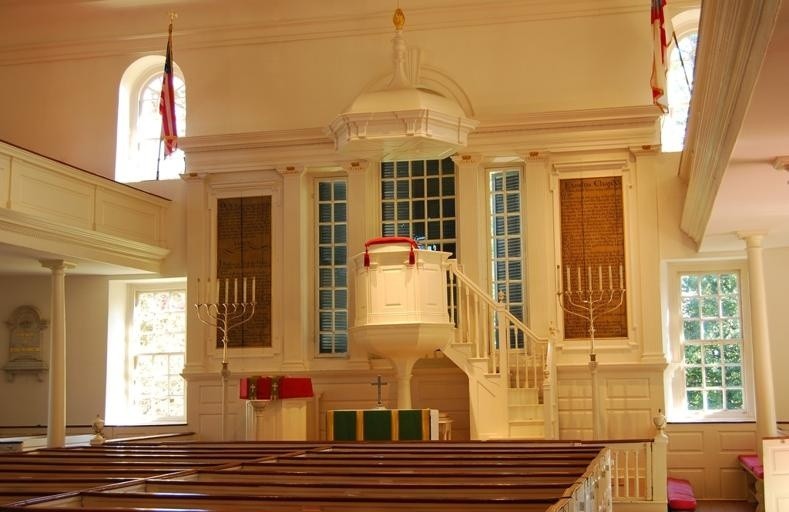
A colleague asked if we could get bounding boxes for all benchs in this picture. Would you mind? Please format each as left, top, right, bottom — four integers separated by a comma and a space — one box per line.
667, 477, 697, 512
0, 438, 614, 512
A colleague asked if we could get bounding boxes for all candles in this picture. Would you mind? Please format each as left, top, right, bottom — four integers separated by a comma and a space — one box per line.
552, 263, 626, 291
195, 277, 256, 303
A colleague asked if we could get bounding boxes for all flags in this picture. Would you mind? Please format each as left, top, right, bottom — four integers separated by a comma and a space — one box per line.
646, 1, 674, 119
157, 20, 179, 161
